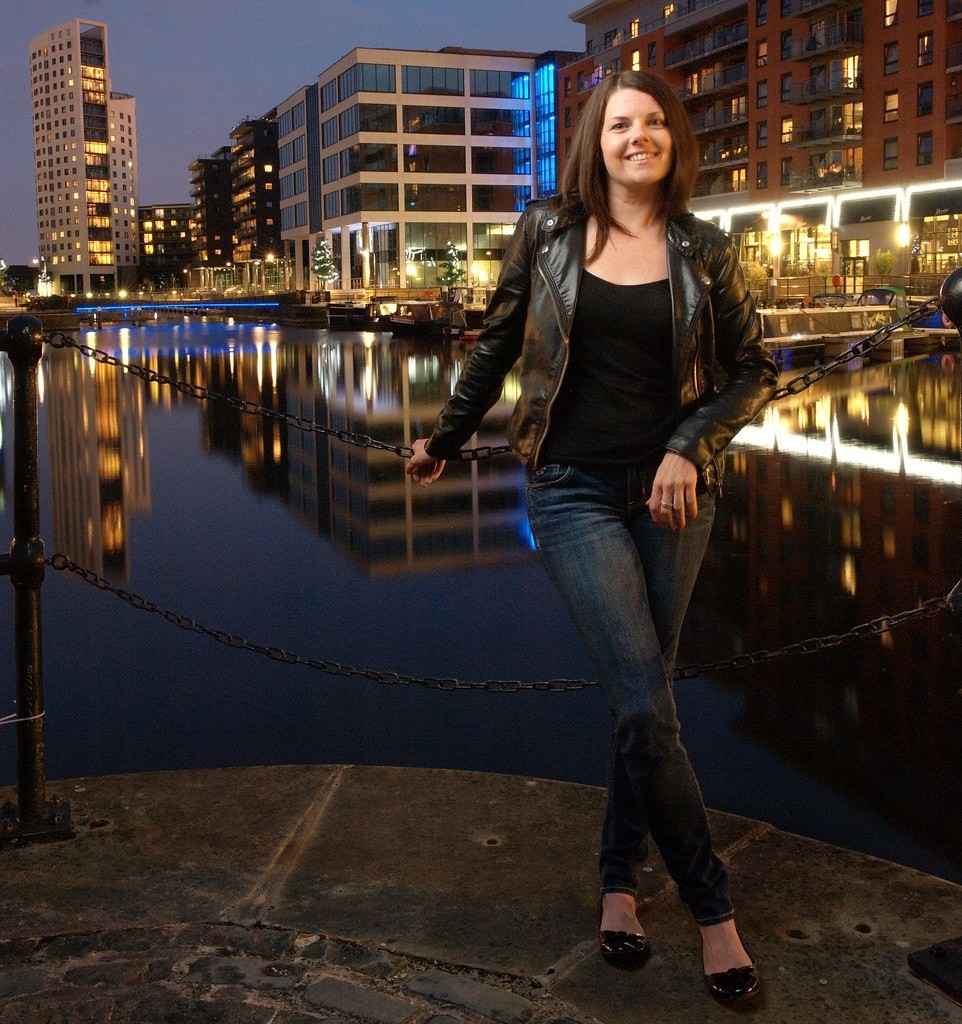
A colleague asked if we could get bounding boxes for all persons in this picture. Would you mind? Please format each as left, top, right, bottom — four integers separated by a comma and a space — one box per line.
406, 70, 779, 999
291, 290, 326, 304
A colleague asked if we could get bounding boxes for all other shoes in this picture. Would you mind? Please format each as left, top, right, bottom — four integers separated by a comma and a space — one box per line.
596, 894, 645, 959
701, 926, 761, 1002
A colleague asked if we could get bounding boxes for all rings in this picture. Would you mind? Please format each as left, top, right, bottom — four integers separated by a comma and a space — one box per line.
661, 501, 673, 509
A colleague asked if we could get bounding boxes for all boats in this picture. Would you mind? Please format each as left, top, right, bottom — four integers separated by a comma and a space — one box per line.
324, 300, 368, 331
388, 300, 466, 340
803, 287, 914, 331
